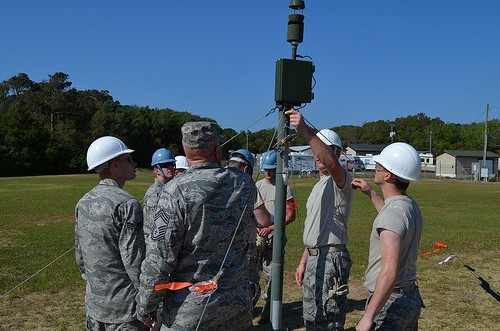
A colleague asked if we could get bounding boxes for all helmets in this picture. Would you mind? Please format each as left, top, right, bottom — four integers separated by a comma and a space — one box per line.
230, 149, 254, 176
316, 129, 343, 150
373, 142, 421, 182
86, 136, 135, 171
261, 150, 277, 169
174, 156, 189, 170
151, 148, 177, 166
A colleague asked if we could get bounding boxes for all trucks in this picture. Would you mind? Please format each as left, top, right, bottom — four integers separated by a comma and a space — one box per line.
338, 155, 365, 171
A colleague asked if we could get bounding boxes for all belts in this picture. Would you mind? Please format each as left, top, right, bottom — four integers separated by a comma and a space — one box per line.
368, 281, 415, 293
307, 245, 345, 255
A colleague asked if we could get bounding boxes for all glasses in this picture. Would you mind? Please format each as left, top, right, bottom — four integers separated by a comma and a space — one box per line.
376, 163, 386, 172
160, 163, 173, 168
125, 156, 132, 162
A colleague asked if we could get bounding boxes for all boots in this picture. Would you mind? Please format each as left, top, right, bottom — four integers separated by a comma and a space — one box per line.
256, 302, 270, 324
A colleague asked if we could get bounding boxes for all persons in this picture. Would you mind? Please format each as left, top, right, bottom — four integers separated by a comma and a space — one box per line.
135, 122, 259, 331
285, 110, 354, 331
174, 156, 189, 177
142, 148, 177, 242
351, 143, 426, 331
253, 150, 296, 322
227, 148, 272, 228
74, 136, 149, 331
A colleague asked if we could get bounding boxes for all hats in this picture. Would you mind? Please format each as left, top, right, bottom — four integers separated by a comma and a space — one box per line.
181, 122, 225, 149
